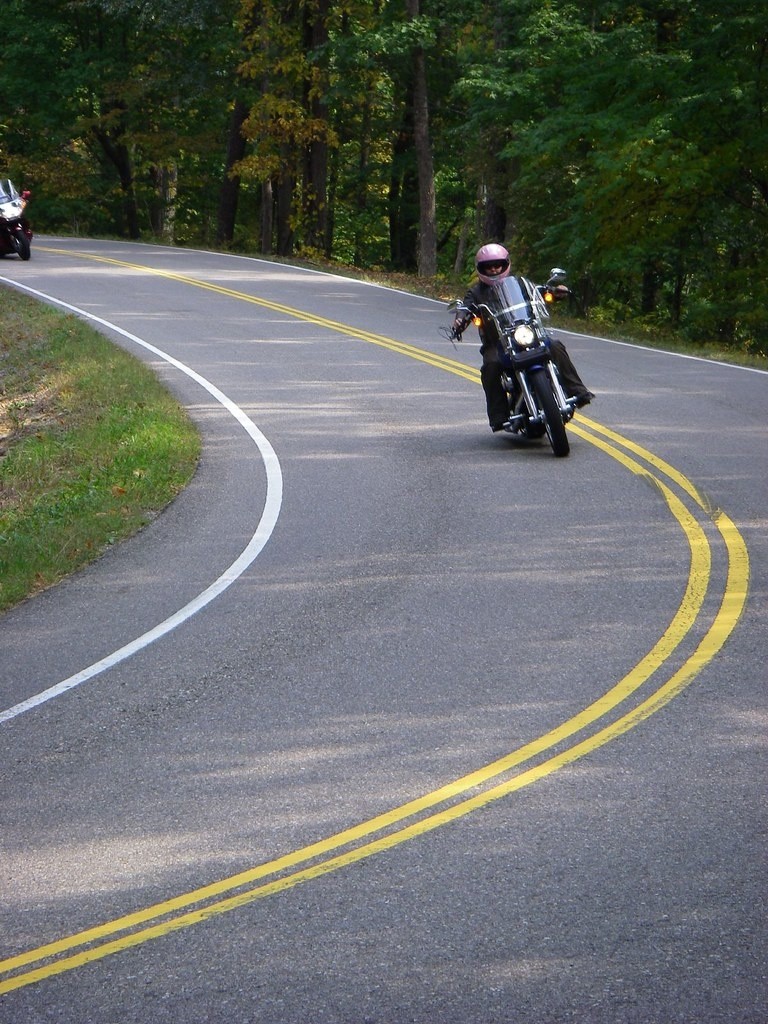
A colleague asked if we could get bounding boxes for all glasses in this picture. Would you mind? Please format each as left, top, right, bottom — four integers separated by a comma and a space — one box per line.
483, 262, 502, 270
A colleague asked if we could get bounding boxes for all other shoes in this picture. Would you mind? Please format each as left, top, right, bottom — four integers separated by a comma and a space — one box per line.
489, 417, 508, 432
575, 391, 592, 409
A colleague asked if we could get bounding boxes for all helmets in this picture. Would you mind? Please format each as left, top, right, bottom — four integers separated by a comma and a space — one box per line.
475, 244, 511, 285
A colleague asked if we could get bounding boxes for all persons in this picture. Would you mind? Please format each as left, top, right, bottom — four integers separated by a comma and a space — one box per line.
453, 244, 590, 429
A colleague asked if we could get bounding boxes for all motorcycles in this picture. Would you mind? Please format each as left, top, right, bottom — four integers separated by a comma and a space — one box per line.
0, 177, 33, 262
447, 268, 591, 458
